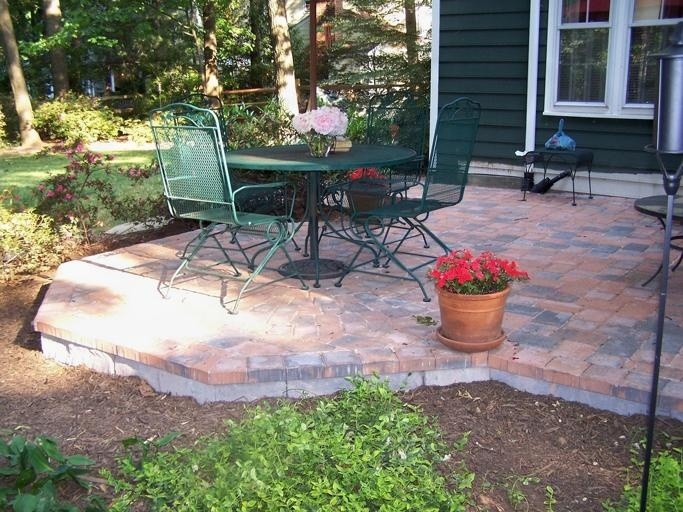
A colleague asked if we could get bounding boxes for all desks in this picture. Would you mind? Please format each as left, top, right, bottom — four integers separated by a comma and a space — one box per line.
635, 195, 683, 287
229, 145, 417, 278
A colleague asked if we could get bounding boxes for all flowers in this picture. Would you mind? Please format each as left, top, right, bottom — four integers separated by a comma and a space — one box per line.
426, 243, 531, 296
293, 105, 351, 135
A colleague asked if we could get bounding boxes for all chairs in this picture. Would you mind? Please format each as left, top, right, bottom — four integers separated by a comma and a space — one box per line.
150, 104, 309, 313
334, 99, 483, 301
311, 92, 428, 267
169, 94, 303, 269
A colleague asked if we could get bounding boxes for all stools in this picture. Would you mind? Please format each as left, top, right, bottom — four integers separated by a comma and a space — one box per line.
518, 148, 593, 205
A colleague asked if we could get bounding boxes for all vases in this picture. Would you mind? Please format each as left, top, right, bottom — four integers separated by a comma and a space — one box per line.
309, 133, 331, 157
434, 288, 510, 353
